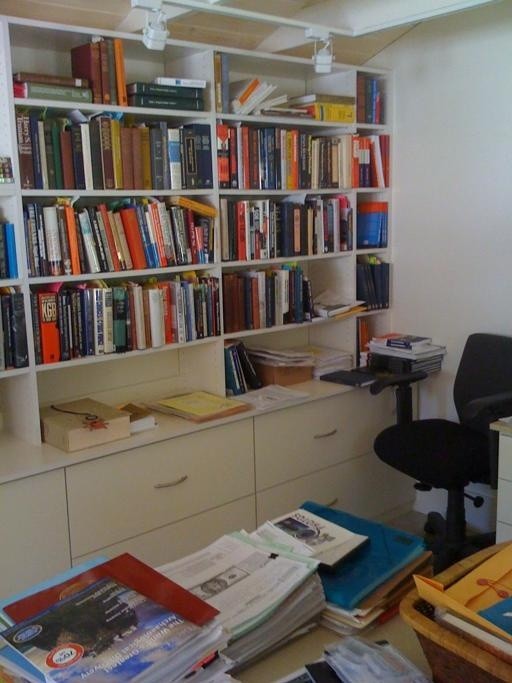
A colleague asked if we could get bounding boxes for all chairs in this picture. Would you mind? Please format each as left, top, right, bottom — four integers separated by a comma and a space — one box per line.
379, 333, 512, 571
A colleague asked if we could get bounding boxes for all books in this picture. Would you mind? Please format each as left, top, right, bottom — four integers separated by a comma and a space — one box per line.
220, 195, 353, 260
1, 293, 29, 369
31, 272, 221, 364
356, 77, 385, 124
0, 500, 434, 683
358, 317, 445, 372
24, 198, 216, 277
216, 124, 390, 189
1, 223, 18, 279
224, 263, 350, 333
357, 202, 388, 249
357, 262, 389, 309
16, 111, 213, 190
214, 53, 354, 122
40, 399, 156, 451
13, 39, 206, 111
222, 338, 353, 395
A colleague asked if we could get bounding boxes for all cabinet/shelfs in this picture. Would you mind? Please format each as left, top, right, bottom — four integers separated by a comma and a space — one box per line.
2, 14, 419, 630
